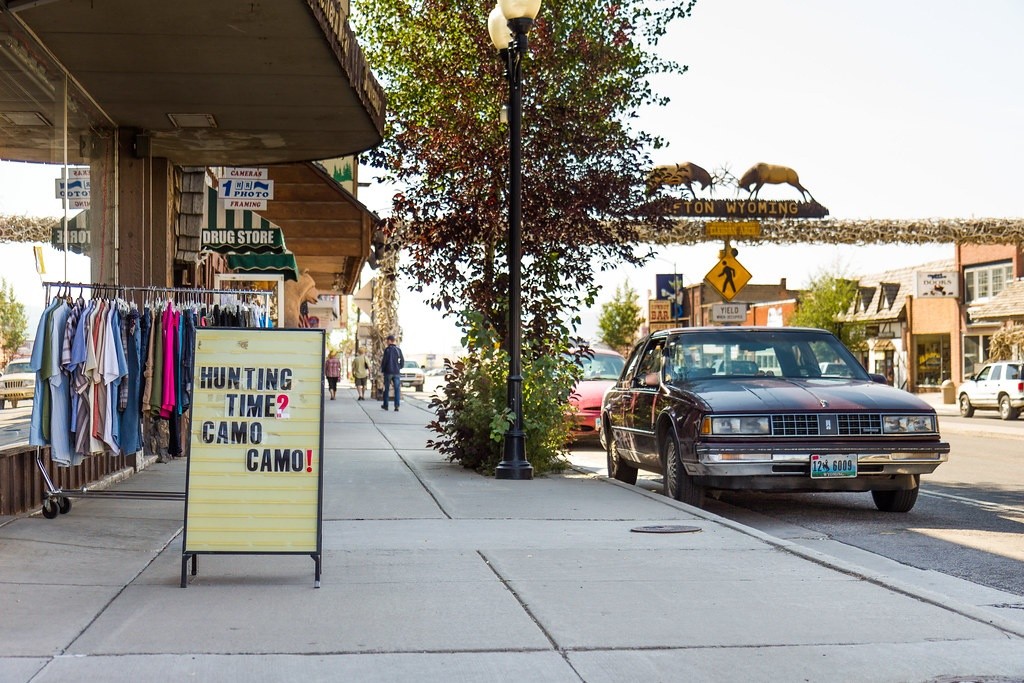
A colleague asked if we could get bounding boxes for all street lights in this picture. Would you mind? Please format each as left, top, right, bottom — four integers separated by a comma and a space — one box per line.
486, 0, 547, 481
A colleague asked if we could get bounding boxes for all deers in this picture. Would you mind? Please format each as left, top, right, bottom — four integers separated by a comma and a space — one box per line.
721, 162, 818, 203
643, 162, 728, 200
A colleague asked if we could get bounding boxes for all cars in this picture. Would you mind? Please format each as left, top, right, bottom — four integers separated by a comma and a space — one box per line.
545, 346, 658, 446
600, 325, 952, 514
396, 360, 426, 392
426, 367, 447, 377
956, 360, 1024, 421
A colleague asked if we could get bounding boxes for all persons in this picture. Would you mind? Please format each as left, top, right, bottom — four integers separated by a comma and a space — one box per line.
325, 350, 341, 399
351, 347, 370, 401
381, 335, 405, 411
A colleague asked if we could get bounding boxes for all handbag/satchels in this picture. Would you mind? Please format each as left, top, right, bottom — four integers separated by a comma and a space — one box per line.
364, 357, 369, 369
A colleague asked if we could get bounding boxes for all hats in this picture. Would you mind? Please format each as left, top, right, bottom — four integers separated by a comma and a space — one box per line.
385, 335, 395, 340
329, 351, 338, 357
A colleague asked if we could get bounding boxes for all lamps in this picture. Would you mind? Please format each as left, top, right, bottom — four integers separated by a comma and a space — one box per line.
333, 274, 341, 281
332, 282, 339, 291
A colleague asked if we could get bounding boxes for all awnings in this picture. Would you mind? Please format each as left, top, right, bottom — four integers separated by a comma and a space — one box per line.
226, 248, 298, 283
203, 183, 286, 255
51, 210, 90, 254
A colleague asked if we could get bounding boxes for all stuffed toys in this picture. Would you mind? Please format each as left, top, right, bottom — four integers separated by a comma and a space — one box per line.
283, 268, 322, 328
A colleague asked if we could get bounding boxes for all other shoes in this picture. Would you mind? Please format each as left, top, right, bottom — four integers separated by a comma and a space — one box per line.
358, 397, 361, 400
381, 405, 388, 410
331, 397, 334, 400
394, 405, 398, 411
362, 397, 365, 400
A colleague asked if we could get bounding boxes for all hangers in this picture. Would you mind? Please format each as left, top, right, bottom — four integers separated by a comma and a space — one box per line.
44, 280, 274, 315
